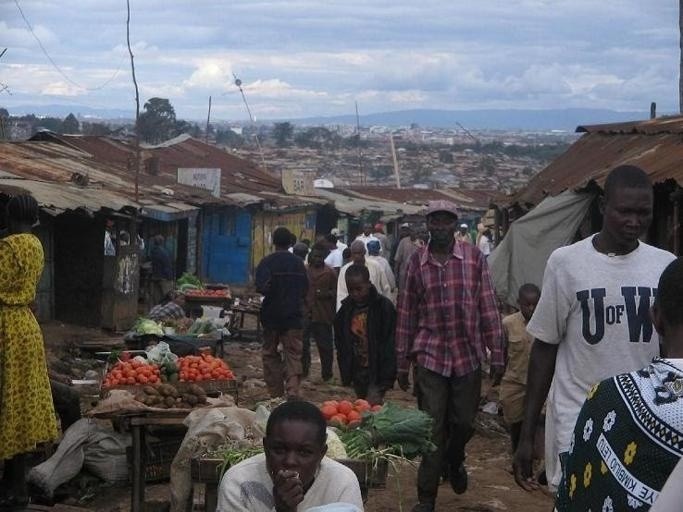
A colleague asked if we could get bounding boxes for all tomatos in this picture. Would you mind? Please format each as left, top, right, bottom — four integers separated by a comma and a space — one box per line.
186, 290, 228, 298
315, 399, 382, 424
102, 352, 162, 386
175, 353, 238, 382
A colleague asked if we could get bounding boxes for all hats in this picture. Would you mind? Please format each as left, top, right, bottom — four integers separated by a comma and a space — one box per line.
424, 199, 460, 220
401, 222, 415, 233
367, 241, 381, 252
374, 223, 384, 232
342, 248, 351, 259
293, 243, 306, 257
330, 227, 344, 238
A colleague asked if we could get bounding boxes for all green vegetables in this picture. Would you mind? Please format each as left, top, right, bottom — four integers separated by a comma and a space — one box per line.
339, 402, 438, 461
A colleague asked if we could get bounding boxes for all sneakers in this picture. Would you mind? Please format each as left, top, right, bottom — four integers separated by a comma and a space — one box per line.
410, 499, 436, 512
447, 466, 469, 496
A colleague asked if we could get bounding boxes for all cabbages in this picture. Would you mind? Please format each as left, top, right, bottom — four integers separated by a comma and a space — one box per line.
136, 320, 161, 335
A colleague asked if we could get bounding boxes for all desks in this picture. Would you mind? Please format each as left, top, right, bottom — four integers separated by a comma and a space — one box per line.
230, 306, 262, 339
192, 448, 388, 510
123, 416, 213, 511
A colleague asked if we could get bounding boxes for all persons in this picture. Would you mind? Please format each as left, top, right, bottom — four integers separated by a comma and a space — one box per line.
512, 166, 677, 501
553, 258, 683, 512
0, 195, 58, 507
150, 234, 173, 306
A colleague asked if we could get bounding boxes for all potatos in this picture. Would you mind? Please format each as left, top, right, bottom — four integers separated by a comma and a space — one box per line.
134, 383, 212, 408
